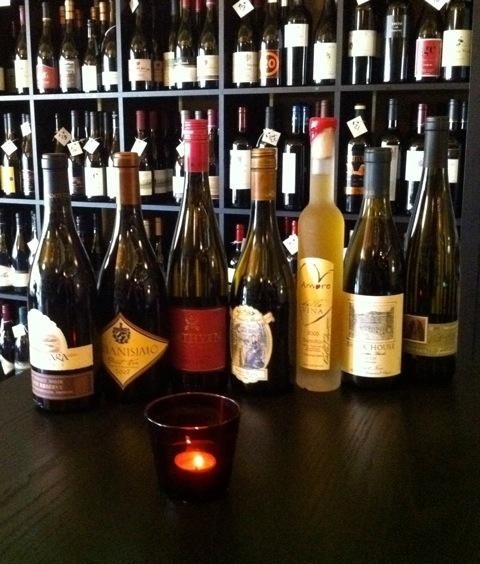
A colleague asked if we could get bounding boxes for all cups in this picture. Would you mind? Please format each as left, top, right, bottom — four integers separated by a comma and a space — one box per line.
144, 391, 240, 495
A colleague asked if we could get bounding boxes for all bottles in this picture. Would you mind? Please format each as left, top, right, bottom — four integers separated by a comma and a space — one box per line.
27, 152, 97, 412
129, 110, 154, 204
228, 224, 247, 293
27, 209, 39, 273
163, 0, 176, 90
35, 2, 59, 93
404, 116, 461, 388
97, 111, 103, 139
75, 7, 85, 47
10, 213, 30, 294
375, 14, 383, 83
89, 213, 105, 276
231, 0, 259, 87
228, 107, 254, 209
254, 106, 279, 210
101, 25, 119, 92
344, 102, 374, 210
230, 147, 294, 395
99, 1, 109, 45
98, 152, 166, 401
415, 0, 442, 83
153, 217, 169, 274
75, 216, 85, 244
0, 304, 14, 380
302, 106, 309, 136
127, 1, 152, 91
258, 0, 282, 87
312, 1, 337, 85
0, 112, 19, 198
191, 0, 203, 36
0, 60, 5, 95
58, 6, 65, 48
281, 216, 290, 240
103, 112, 111, 153
314, 100, 320, 117
404, 102, 427, 216
344, 230, 354, 260
446, 97, 466, 216
195, 109, 202, 120
84, 112, 106, 202
160, 110, 175, 201
172, 109, 193, 205
207, 109, 219, 208
16, 5, 29, 95
294, 118, 345, 392
279, 0, 289, 25
81, 19, 101, 93
0, 212, 12, 293
152, 6, 163, 91
67, 110, 84, 201
282, 0, 312, 86
165, 118, 230, 397
143, 219, 152, 248
18, 113, 35, 199
4, 114, 7, 143
59, 0, 81, 93
442, 1, 472, 81
349, 0, 377, 85
176, 0, 197, 89
197, 0, 220, 89
148, 111, 169, 203
376, 98, 403, 215
284, 220, 299, 278
343, 148, 407, 390
5, 20, 16, 94
90, 6, 99, 47
80, 110, 89, 142
106, 110, 119, 202
170, 112, 181, 139
54, 112, 64, 153
278, 104, 309, 211
320, 99, 330, 117
109, 0, 115, 29
14, 307, 30, 374
382, 0, 409, 84
